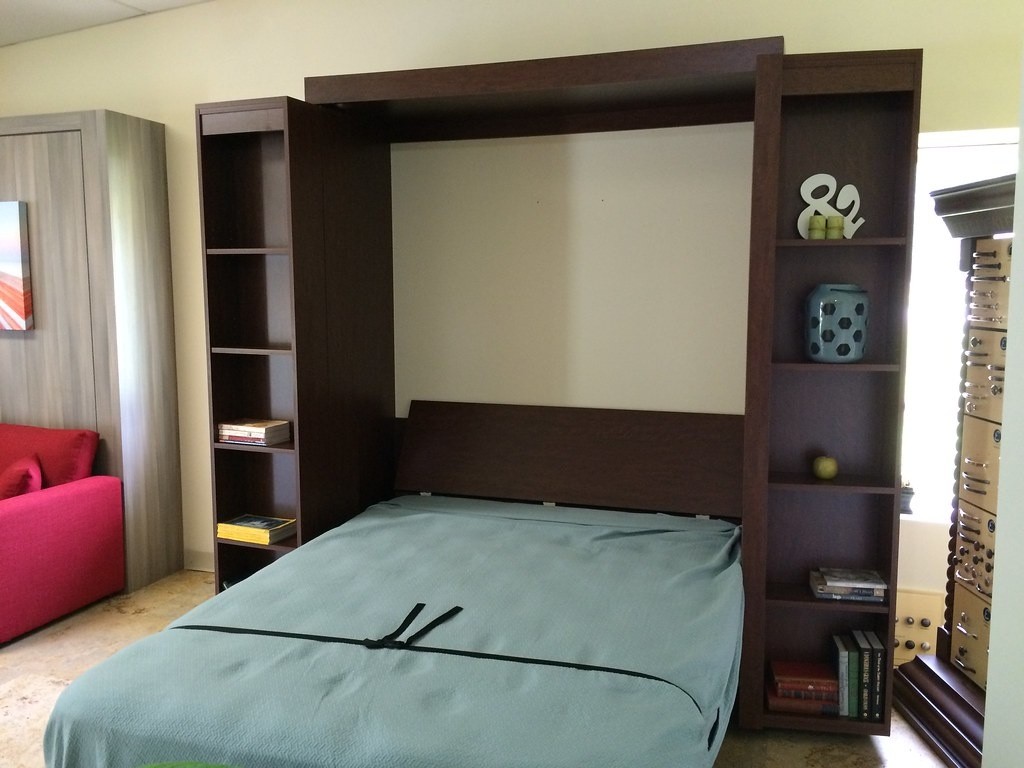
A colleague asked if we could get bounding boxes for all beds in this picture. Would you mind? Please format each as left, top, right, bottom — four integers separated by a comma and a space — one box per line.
44, 493, 745, 768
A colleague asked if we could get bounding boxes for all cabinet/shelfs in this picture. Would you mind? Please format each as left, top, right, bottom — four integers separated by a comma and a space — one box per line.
949, 234, 1012, 688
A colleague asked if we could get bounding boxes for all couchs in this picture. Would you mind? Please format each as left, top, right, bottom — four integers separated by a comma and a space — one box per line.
0, 422, 125, 642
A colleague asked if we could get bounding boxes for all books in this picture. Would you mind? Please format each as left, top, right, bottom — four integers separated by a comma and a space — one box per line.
763, 562, 891, 725
213, 416, 299, 547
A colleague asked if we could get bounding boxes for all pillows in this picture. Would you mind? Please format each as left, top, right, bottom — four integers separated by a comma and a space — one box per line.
1, 451, 42, 499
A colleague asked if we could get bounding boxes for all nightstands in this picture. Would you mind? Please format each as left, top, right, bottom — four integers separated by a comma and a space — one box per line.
195, 96, 300, 596
753, 49, 921, 736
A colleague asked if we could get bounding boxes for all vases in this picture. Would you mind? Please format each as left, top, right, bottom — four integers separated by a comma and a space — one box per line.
804, 282, 871, 363
